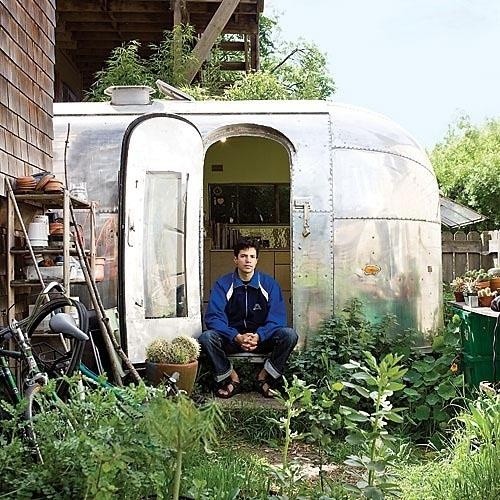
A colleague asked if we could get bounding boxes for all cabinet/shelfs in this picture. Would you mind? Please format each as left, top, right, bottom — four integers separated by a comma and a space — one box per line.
210, 182, 293, 328
10, 190, 97, 313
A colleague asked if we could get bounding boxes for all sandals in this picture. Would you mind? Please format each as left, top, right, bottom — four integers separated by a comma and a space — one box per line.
256, 376, 276, 398
217, 378, 242, 399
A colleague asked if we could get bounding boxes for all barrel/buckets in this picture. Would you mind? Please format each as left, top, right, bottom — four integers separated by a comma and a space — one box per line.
88, 256, 106, 283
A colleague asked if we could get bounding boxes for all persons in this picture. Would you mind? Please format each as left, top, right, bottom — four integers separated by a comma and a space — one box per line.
199, 236, 298, 398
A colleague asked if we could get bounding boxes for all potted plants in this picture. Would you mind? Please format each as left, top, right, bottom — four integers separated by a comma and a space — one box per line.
145, 336, 199, 396
450, 268, 500, 307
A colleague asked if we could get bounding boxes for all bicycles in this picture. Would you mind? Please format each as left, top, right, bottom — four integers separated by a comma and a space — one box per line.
0, 277, 184, 482
